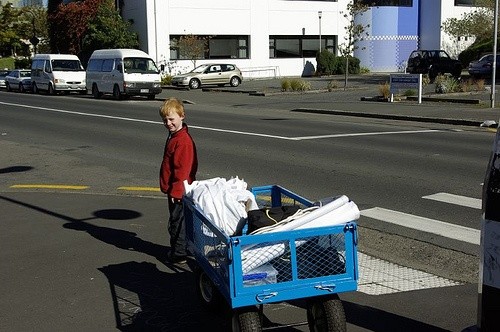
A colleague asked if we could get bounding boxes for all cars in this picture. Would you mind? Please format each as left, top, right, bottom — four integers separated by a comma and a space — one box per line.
0, 69, 12, 88
469, 54, 500, 77
171, 63, 243, 90
4, 69, 31, 92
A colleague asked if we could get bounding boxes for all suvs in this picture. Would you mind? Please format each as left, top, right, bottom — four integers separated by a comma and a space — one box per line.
406, 49, 463, 76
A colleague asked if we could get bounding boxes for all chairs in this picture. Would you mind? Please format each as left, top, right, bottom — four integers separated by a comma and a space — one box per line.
138, 64, 147, 70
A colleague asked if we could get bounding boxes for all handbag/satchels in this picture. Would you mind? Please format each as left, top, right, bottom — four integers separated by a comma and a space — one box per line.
247, 205, 301, 235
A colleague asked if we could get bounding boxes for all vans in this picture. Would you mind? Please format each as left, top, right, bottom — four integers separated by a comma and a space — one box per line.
86, 48, 165, 100
31, 54, 87, 96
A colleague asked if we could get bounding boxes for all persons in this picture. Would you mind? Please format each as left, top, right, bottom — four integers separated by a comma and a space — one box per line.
158, 96, 198, 263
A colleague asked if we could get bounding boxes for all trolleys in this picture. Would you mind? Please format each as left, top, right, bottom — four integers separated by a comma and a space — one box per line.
179, 185, 359, 332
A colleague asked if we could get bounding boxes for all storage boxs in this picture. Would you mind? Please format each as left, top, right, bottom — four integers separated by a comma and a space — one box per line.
216, 257, 279, 289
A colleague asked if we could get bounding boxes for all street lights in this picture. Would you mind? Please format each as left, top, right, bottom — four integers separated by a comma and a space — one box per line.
318, 10, 323, 54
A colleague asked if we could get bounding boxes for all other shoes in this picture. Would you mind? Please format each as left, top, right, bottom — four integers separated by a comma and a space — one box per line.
186, 253, 196, 261
160, 255, 187, 264
